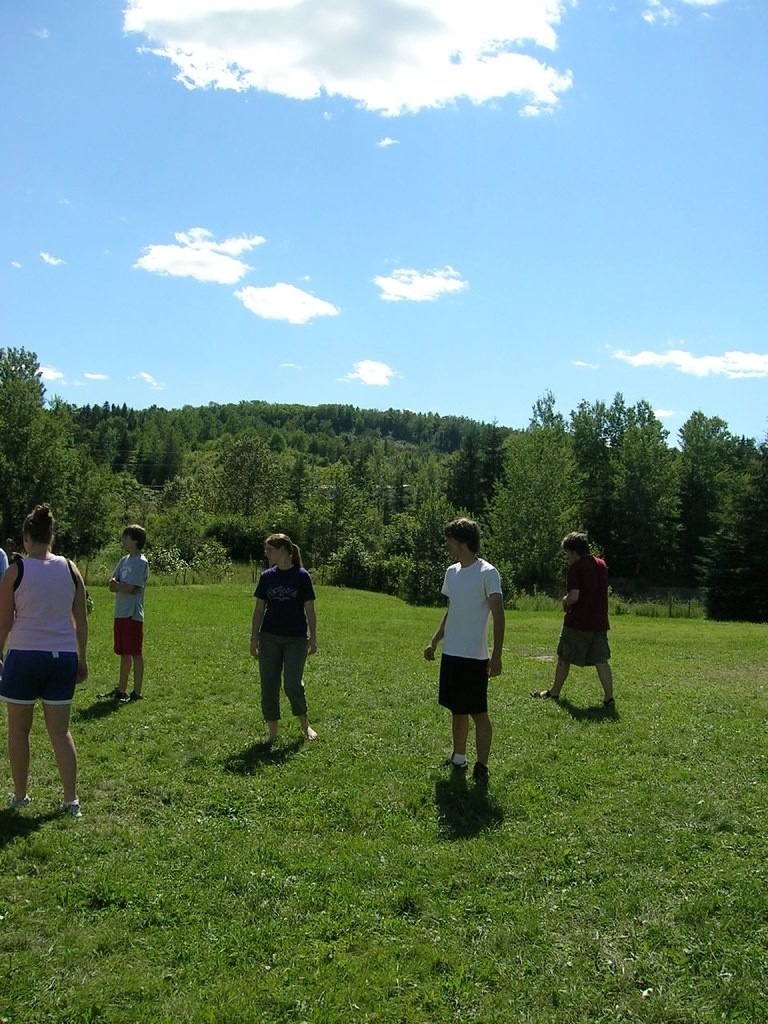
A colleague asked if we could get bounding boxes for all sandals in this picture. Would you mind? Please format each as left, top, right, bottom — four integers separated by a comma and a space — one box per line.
530, 690, 559, 700
602, 698, 615, 709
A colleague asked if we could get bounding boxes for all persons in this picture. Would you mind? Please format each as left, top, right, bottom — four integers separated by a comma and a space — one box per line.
0, 504, 89, 818
250, 534, 319, 741
96, 524, 144, 701
529, 532, 616, 706
423, 517, 505, 778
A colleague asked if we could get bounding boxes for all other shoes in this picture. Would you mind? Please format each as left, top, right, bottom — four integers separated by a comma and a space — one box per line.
468, 764, 489, 784
11, 794, 30, 808
96, 688, 127, 702
120, 690, 143, 704
427, 751, 468, 772
59, 802, 82, 818
